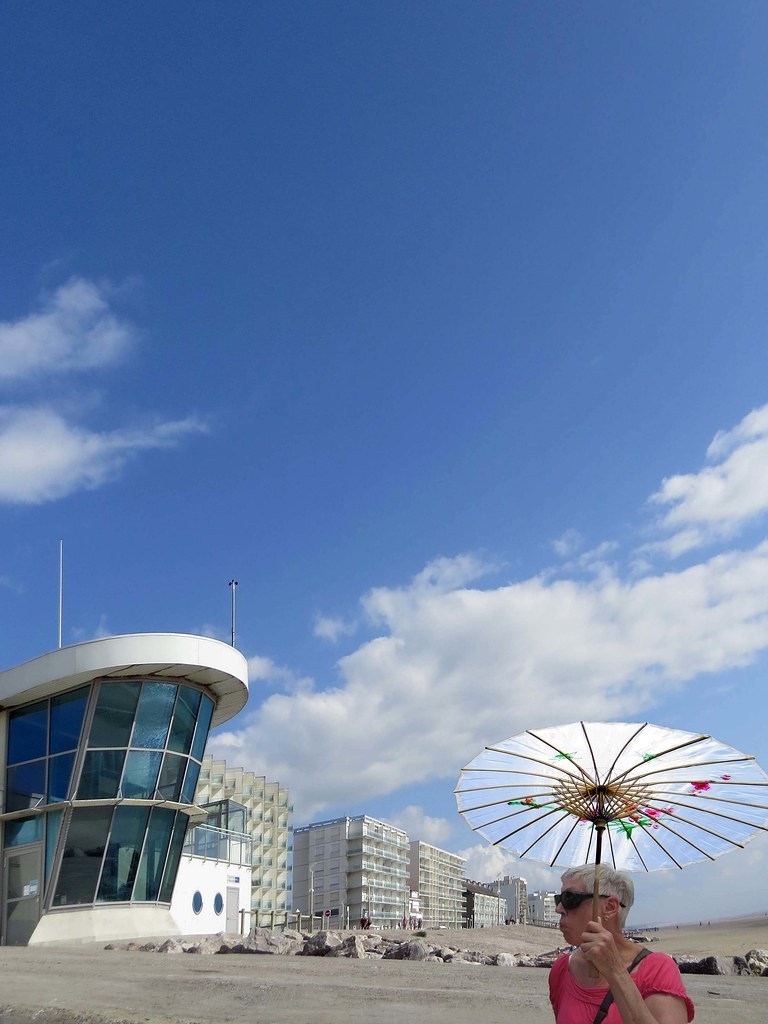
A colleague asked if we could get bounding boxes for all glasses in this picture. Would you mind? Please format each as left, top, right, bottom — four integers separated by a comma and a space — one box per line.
554, 890, 626, 910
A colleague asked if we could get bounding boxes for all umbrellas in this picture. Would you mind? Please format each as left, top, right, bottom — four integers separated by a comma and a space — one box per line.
453, 720, 768, 978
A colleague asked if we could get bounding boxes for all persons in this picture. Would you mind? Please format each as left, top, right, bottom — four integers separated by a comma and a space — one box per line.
505, 919, 508, 925
418, 918, 422, 930
549, 863, 695, 1024
414, 919, 418, 930
360, 915, 366, 929
409, 916, 414, 930
557, 947, 560, 955
403, 917, 408, 930
569, 945, 576, 953
510, 915, 515, 924
